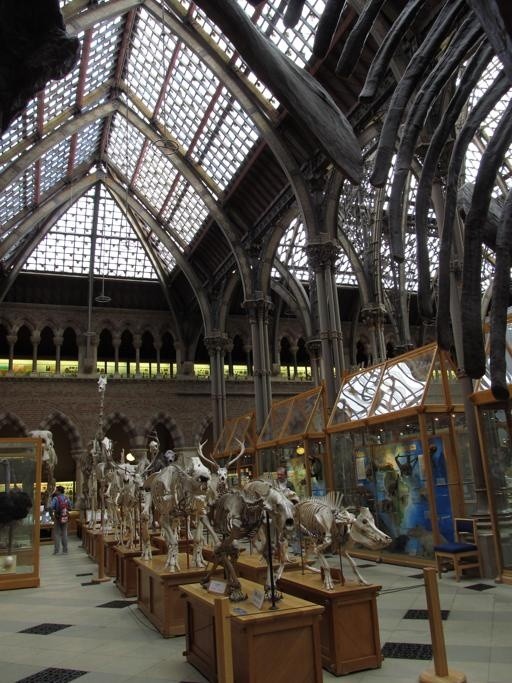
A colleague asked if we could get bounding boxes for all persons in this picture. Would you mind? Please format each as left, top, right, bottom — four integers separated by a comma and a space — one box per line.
273, 465, 302, 557
49, 485, 73, 555
362, 467, 377, 497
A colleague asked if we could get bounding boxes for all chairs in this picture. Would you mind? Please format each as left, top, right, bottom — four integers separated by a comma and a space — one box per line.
434, 518, 485, 583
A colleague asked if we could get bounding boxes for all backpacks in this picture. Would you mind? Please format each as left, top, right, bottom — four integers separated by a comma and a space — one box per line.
58, 496, 70, 524
